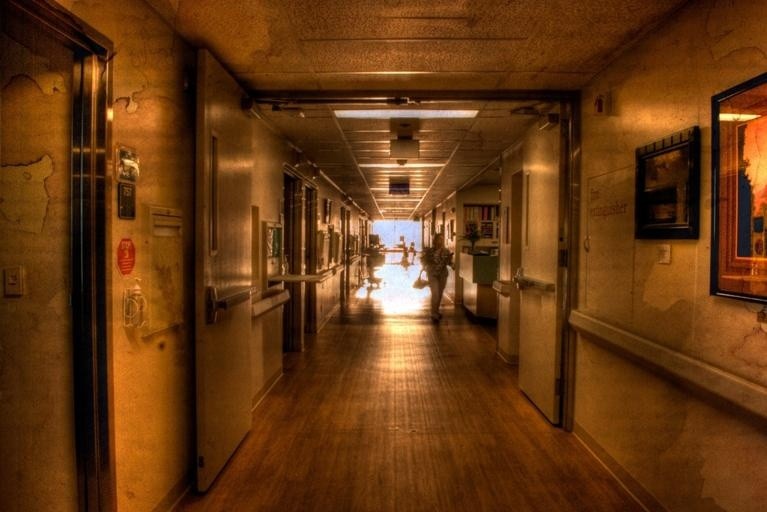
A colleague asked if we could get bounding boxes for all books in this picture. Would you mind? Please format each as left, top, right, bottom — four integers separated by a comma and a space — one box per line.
465, 206, 499, 239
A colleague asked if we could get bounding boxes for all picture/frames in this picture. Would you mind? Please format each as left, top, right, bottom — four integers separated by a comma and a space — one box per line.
633, 73, 767, 305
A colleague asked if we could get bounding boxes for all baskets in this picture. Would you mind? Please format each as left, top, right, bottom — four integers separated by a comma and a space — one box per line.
413, 269, 430, 289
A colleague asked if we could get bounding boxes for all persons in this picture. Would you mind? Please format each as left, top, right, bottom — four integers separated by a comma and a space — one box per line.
421, 234, 456, 324
